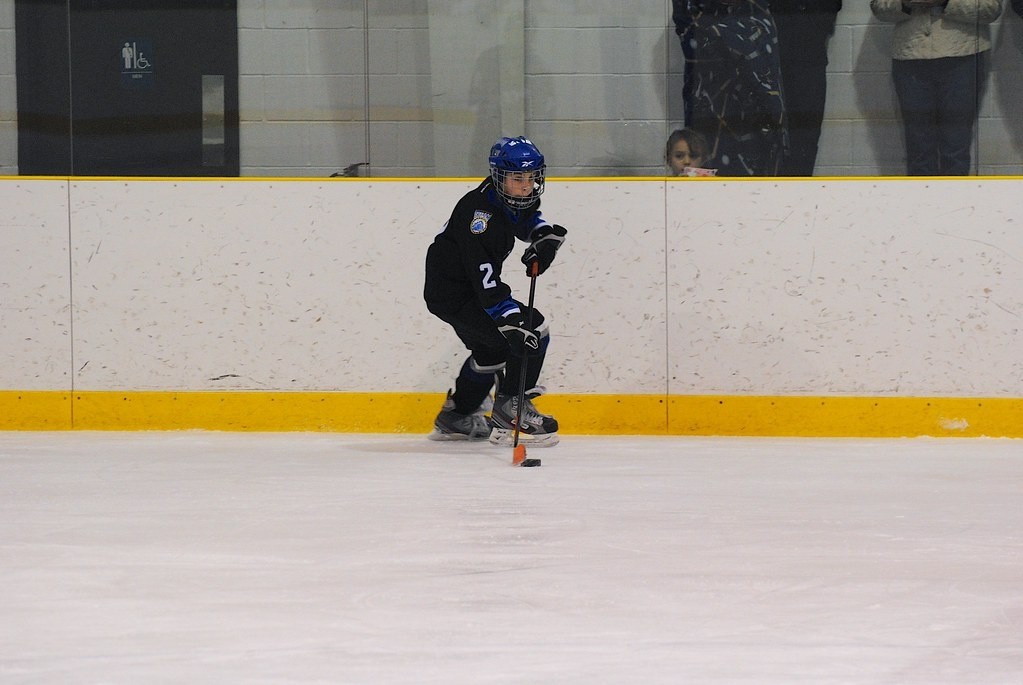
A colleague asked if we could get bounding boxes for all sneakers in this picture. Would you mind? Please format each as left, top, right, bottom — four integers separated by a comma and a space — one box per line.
492, 370, 558, 433
433, 388, 492, 439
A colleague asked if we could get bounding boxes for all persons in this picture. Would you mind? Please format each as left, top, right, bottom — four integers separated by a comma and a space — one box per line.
765, 0, 841, 178
663, 130, 715, 180
422, 135, 568, 447
868, 0, 1004, 175
671, 0, 792, 178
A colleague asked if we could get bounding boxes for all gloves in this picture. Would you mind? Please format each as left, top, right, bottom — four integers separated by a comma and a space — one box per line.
521, 224, 567, 277
484, 292, 542, 355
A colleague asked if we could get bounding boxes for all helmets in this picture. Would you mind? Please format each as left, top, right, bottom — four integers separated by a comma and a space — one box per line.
489, 135, 547, 210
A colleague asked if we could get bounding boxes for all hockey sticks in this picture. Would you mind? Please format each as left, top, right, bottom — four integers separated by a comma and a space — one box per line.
512, 258, 542, 467
